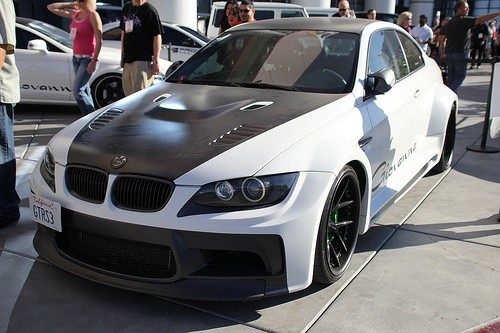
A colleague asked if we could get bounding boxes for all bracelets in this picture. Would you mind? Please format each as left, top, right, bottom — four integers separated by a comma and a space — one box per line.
92, 58, 99, 61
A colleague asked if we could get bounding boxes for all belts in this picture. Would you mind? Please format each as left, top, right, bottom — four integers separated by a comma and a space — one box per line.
74, 55, 93, 60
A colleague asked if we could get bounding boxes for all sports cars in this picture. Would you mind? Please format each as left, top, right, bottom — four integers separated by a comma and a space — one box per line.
27, 17, 460, 302
101, 20, 225, 78
14, 17, 172, 110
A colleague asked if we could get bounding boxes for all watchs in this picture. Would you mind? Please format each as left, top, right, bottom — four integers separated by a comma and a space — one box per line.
153, 60, 159, 64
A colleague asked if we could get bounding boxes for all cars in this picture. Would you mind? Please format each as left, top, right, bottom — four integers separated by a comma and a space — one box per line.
197, 0, 400, 55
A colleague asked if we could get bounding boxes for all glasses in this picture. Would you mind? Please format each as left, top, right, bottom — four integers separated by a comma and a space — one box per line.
240, 9, 250, 13
339, 8, 346, 11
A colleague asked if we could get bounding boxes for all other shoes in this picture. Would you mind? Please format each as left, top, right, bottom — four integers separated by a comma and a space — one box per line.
470, 65, 476, 69
477, 66, 481, 69
0, 213, 21, 230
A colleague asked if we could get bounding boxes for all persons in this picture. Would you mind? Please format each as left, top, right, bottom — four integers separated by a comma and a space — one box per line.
0, 0, 21, 229
119, 0, 165, 96
389, 11, 423, 79
367, 9, 376, 20
412, 14, 500, 76
216, 0, 258, 71
339, 0, 356, 18
439, 0, 500, 91
47, 0, 102, 116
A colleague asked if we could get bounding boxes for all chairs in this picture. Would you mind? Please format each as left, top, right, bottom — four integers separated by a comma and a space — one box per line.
306, 45, 330, 69
350, 48, 390, 80
